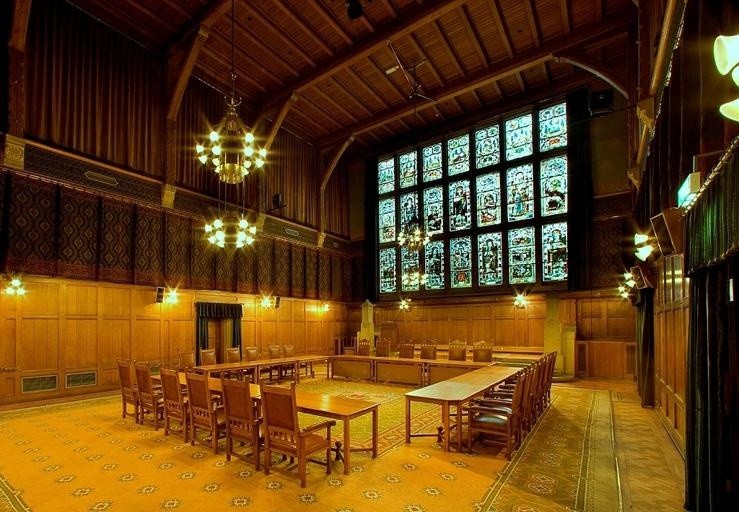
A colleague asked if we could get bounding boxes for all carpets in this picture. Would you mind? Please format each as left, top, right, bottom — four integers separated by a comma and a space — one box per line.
1, 374, 623, 511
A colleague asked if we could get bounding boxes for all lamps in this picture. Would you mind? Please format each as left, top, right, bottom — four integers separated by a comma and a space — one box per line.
195, 1, 268, 185
677, 145, 728, 209
205, 177, 258, 251
398, 66, 430, 287
714, 32, 739, 124
617, 206, 685, 306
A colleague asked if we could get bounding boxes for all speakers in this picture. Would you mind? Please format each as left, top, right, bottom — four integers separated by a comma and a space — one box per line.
155, 287, 165, 303
274, 296, 280, 308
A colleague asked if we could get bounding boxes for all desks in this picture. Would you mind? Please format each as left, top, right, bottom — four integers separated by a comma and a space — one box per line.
149, 371, 379, 477
190, 355, 337, 397
332, 354, 497, 389
403, 362, 524, 448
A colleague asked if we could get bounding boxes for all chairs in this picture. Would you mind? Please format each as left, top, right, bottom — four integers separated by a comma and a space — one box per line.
357, 337, 492, 363
177, 343, 307, 381
455, 350, 562, 462
117, 357, 337, 488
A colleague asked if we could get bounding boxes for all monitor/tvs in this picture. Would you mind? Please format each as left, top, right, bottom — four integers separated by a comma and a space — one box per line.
629, 263, 655, 291
649, 209, 684, 257
